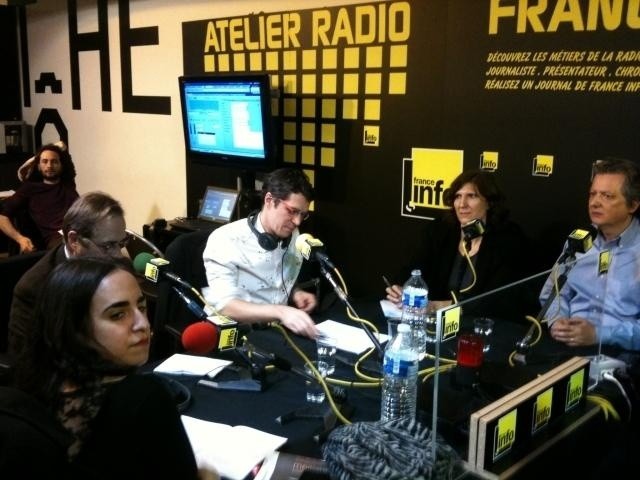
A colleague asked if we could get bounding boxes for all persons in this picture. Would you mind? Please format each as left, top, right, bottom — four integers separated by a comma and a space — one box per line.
202, 162, 326, 342
0, 143, 79, 256
7, 190, 139, 400
384, 166, 541, 321
539, 161, 640, 391
18, 252, 223, 480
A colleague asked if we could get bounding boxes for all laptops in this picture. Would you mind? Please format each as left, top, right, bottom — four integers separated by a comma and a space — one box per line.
169, 185, 241, 232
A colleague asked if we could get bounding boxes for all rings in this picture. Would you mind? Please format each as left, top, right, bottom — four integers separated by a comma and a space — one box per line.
568, 337, 574, 343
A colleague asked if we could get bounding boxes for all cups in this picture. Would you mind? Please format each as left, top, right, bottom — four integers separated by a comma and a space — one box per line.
303, 361, 328, 403
456, 333, 485, 368
317, 336, 337, 375
472, 316, 495, 355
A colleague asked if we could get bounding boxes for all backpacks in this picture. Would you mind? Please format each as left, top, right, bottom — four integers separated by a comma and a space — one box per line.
321, 416, 465, 480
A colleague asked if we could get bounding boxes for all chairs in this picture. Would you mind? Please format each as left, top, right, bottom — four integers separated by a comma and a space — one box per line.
165, 227, 218, 325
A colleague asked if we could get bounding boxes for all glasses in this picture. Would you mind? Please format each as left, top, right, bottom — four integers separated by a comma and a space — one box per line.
88, 236, 131, 255
272, 196, 310, 221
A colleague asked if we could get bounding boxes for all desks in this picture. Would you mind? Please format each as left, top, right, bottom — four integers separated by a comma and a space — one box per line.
151, 294, 640, 478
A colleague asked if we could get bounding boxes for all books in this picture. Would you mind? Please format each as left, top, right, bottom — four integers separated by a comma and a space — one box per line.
312, 318, 392, 354
153, 351, 235, 380
380, 298, 453, 324
180, 411, 291, 480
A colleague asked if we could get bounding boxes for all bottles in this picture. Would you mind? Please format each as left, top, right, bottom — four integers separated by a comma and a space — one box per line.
400, 269, 428, 362
378, 324, 418, 426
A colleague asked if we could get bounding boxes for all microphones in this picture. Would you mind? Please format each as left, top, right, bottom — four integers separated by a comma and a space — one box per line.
181, 315, 270, 355
294, 233, 336, 270
558, 224, 598, 264
132, 252, 193, 289
461, 217, 487, 241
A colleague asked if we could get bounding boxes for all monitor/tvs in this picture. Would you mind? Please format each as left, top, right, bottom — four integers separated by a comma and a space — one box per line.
177, 71, 273, 171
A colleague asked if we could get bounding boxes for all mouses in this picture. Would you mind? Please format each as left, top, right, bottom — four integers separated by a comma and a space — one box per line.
151, 218, 167, 228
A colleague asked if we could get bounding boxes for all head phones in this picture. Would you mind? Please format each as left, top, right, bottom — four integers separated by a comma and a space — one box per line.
247, 208, 292, 251
